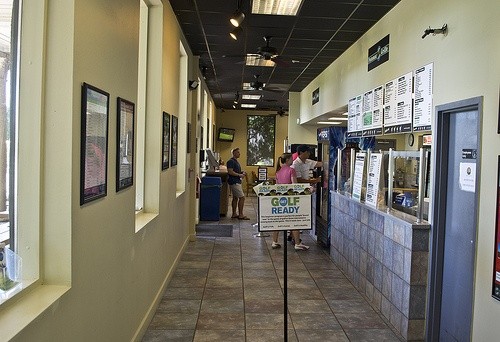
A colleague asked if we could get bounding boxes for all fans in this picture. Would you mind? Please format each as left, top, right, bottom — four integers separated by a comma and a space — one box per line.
234, 74, 289, 93
251, 94, 278, 104
265, 104, 289, 117
221, 35, 301, 65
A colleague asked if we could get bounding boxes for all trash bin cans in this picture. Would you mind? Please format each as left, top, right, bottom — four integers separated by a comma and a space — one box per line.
200, 176, 223, 221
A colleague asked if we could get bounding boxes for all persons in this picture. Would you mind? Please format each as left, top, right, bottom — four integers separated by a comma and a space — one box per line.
227, 147, 250, 220
272, 143, 322, 250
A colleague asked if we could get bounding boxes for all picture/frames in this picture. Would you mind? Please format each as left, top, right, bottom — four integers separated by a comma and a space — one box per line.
162, 111, 180, 170
79, 82, 110, 206
116, 97, 135, 193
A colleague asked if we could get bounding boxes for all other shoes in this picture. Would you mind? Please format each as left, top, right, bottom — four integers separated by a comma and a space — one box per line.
288, 235, 302, 242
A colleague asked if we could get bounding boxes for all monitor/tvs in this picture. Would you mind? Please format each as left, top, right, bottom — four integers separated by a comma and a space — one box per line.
218, 127, 235, 142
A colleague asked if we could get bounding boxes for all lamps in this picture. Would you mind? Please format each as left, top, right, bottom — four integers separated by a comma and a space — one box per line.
234, 91, 240, 104
230, 26, 242, 40
233, 102, 242, 109
229, 11, 245, 28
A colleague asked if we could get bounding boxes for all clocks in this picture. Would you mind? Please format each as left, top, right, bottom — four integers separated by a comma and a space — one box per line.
408, 134, 414, 146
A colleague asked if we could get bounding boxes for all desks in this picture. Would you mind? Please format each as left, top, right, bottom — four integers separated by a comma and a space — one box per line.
256, 174, 277, 184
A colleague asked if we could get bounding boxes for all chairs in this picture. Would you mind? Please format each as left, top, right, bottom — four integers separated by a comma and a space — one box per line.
245, 171, 260, 195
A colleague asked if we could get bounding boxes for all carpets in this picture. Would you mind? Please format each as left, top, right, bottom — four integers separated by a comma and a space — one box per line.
196, 222, 234, 237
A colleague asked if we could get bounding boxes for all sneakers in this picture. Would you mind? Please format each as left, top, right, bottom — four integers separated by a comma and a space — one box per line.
272, 241, 281, 249
295, 244, 310, 250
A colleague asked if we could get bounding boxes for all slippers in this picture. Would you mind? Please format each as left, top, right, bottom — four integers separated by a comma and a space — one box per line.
231, 215, 250, 220
